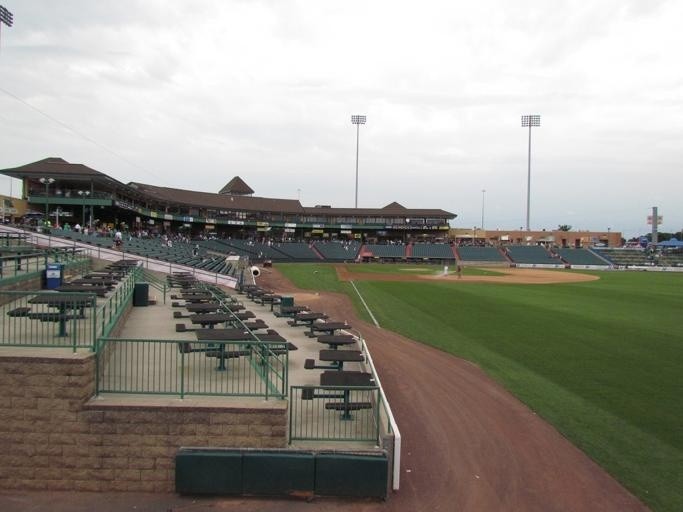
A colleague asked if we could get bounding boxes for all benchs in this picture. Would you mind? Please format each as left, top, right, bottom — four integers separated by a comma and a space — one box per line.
325, 402, 372, 410
168, 282, 183, 288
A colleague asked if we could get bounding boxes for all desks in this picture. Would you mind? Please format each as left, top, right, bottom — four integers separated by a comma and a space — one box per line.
262, 294, 280, 312
319, 350, 363, 371
86, 273, 111, 279
296, 312, 327, 327
180, 289, 207, 295
173, 272, 190, 274
281, 306, 310, 313
173, 274, 192, 276
190, 314, 236, 346
174, 276, 194, 280
312, 322, 351, 335
185, 304, 220, 314
243, 287, 260, 299
319, 370, 375, 421
28, 294, 94, 338
251, 291, 272, 301
184, 295, 215, 304
318, 335, 356, 349
176, 279, 199, 285
73, 278, 110, 284
55, 285, 107, 292
239, 284, 256, 293
196, 328, 251, 371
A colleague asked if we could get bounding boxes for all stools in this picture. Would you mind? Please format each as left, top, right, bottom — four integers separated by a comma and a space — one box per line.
300, 383, 344, 400
206, 350, 250, 359
304, 358, 339, 370
172, 302, 185, 307
304, 330, 317, 338
287, 320, 311, 327
167, 275, 174, 278
274, 312, 296, 317
272, 302, 281, 305
167, 278, 177, 281
171, 295, 185, 300
176, 324, 198, 332
6, 306, 58, 318
174, 312, 191, 318
179, 342, 220, 354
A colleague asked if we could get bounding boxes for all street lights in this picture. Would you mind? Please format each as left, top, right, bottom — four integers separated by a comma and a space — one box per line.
351, 114, 366, 208
521, 114, 541, 231
